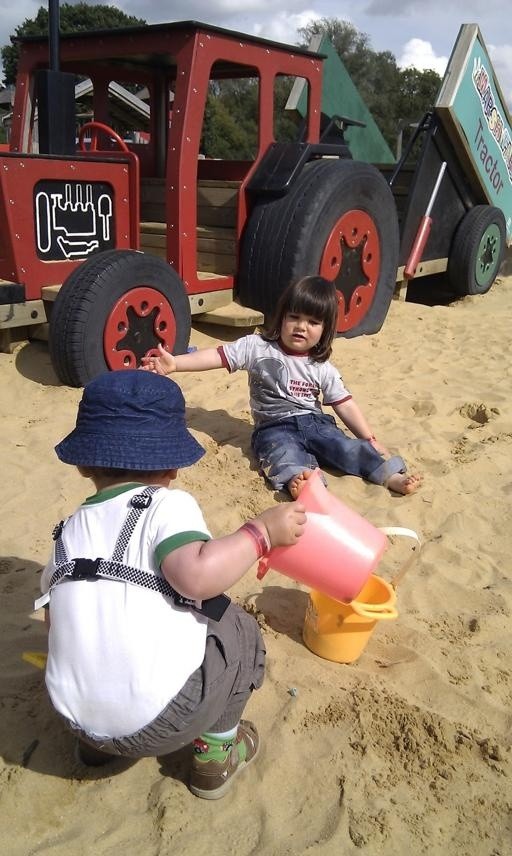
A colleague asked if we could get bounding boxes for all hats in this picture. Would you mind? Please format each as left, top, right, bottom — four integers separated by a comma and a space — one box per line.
53, 369, 208, 472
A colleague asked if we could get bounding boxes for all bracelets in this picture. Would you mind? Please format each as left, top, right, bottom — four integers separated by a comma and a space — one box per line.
366, 437, 376, 442
238, 522, 270, 560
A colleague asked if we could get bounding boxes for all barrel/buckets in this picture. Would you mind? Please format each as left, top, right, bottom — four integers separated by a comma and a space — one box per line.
256, 468, 388, 604
302, 526, 421, 664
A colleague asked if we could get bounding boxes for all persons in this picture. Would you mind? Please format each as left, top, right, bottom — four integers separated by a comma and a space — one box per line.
33, 368, 307, 800
141, 274, 418, 501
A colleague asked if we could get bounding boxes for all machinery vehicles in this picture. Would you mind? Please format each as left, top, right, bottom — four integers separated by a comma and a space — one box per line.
0, 0, 512, 398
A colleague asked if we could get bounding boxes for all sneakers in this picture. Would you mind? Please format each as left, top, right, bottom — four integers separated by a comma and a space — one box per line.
188, 719, 261, 801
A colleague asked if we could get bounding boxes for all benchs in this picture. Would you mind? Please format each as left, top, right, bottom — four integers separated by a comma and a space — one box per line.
138, 176, 242, 275
